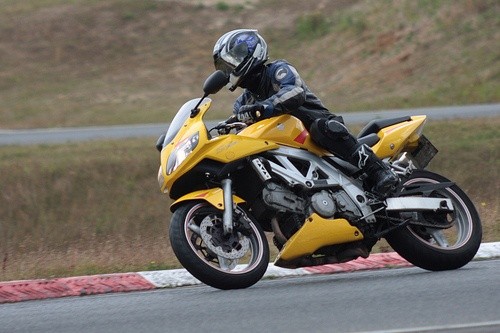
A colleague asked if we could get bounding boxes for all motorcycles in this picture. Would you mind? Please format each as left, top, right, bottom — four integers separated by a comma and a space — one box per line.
154, 69, 483, 290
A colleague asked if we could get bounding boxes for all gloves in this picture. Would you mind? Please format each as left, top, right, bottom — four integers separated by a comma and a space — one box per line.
237, 104, 263, 122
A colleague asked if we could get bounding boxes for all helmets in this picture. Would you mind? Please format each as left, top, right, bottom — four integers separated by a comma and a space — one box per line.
212, 29, 270, 91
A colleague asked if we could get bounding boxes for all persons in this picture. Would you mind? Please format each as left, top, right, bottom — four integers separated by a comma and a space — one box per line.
157, 29, 400, 197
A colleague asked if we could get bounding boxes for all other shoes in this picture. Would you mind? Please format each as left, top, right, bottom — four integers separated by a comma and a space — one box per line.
368, 158, 396, 190
